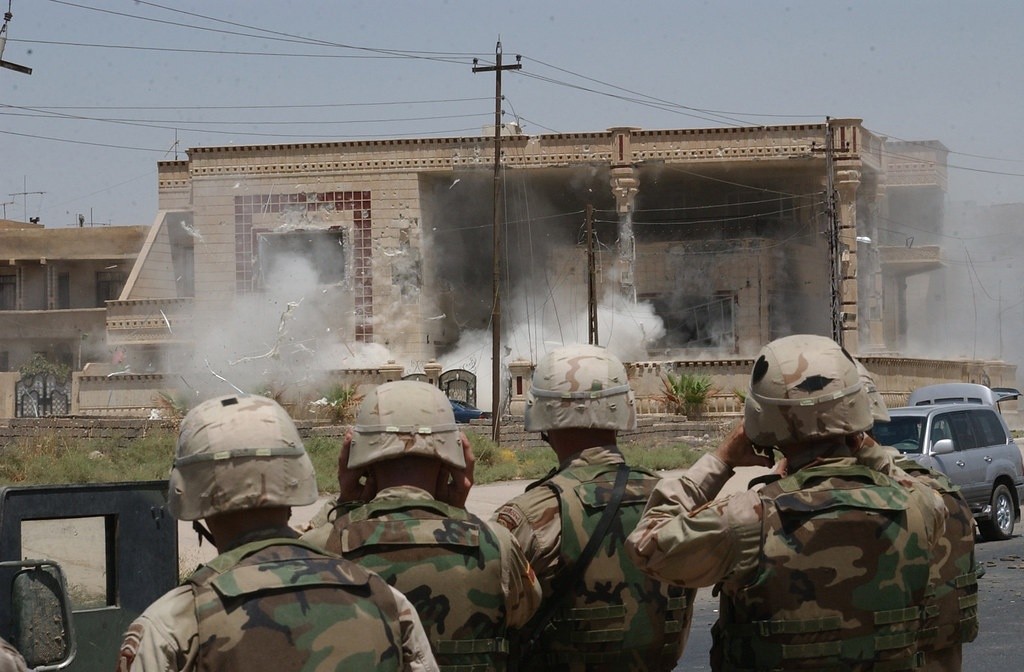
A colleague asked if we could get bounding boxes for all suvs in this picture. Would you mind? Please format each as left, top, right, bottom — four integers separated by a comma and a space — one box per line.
867, 381, 1024, 540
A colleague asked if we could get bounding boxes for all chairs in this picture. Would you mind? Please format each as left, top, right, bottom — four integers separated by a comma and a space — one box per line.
932, 429, 945, 444
955, 420, 973, 448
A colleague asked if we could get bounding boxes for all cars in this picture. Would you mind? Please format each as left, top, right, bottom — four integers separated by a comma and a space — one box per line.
449, 399, 491, 424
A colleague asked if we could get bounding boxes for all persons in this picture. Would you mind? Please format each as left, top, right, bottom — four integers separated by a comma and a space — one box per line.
118, 394, 440, 672
297, 380, 542, 672
487, 342, 698, 672
626, 334, 979, 672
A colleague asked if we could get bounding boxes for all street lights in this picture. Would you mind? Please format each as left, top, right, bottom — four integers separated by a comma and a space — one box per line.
830, 232, 873, 345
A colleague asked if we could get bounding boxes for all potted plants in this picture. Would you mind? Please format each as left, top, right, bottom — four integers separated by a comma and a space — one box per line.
727, 384, 750, 413
655, 370, 727, 421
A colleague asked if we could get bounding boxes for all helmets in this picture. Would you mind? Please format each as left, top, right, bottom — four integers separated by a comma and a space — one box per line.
347, 383, 466, 470
743, 331, 873, 445
850, 353, 892, 426
166, 396, 319, 522
524, 346, 638, 433
